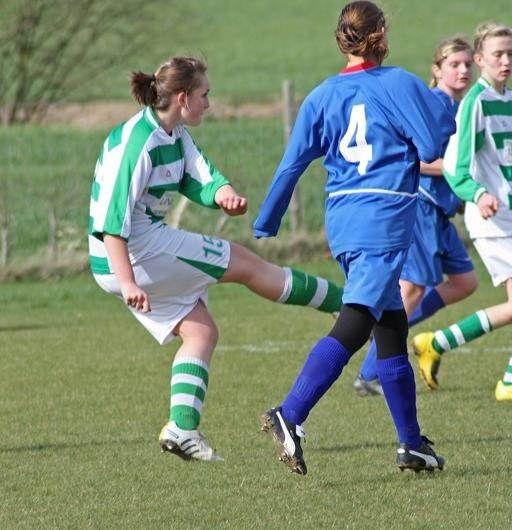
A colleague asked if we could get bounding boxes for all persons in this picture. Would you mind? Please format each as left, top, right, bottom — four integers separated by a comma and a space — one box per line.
411, 21, 512, 401
354, 37, 478, 397
252, 1, 457, 473
88, 57, 343, 462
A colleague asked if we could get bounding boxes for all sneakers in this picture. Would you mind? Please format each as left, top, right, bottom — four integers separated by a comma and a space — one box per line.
494, 378, 512, 402
395, 437, 446, 472
354, 372, 386, 397
259, 406, 307, 477
157, 420, 225, 462
412, 332, 442, 391
